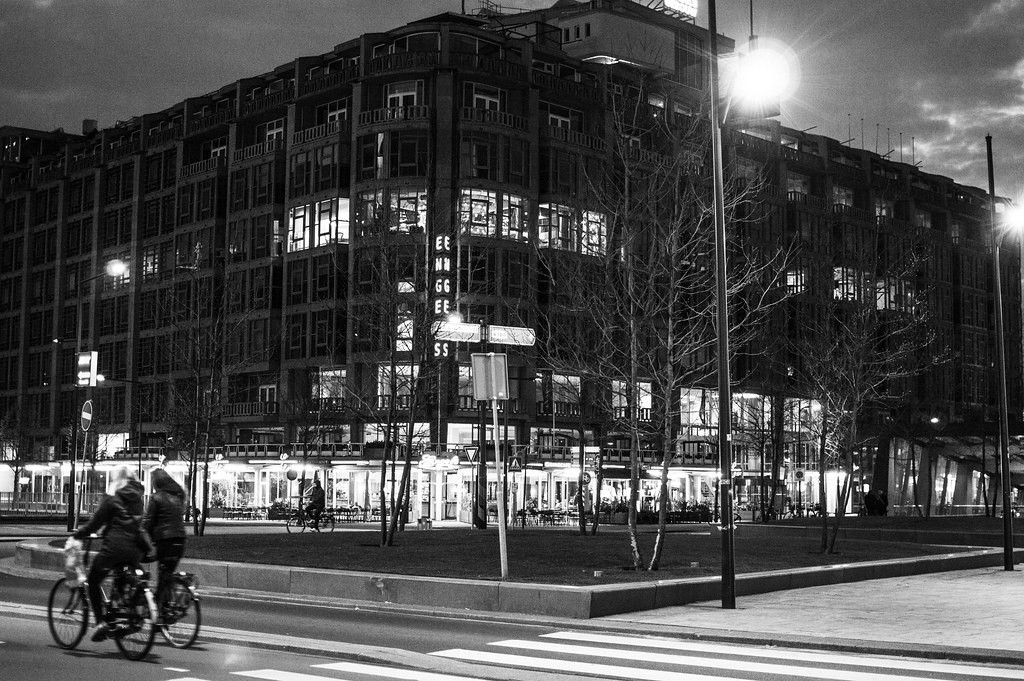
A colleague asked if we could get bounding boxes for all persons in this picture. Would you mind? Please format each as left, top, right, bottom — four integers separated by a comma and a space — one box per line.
71, 467, 186, 642
348, 500, 362, 511
306, 480, 325, 532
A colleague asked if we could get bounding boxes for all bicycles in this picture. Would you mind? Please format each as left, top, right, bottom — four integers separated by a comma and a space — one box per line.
285, 500, 335, 534
47, 535, 203, 661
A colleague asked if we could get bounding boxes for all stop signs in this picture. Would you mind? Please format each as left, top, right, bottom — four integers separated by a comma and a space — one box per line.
80, 400, 94, 432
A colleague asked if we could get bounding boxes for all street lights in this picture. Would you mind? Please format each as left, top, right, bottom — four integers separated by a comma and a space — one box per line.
985, 131, 1024, 572
702, 1, 799, 610
65, 258, 127, 533
95, 373, 143, 482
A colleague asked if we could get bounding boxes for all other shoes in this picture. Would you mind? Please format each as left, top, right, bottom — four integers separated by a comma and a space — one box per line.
91, 622, 110, 642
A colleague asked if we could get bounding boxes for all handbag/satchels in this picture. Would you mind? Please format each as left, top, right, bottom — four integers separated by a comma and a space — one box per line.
64, 536, 88, 588
138, 529, 152, 553
